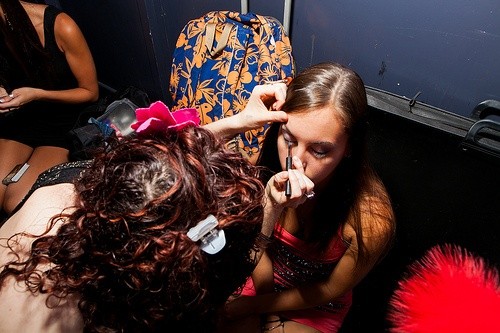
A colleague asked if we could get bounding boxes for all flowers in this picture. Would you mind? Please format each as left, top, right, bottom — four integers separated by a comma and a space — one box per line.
130, 101, 200, 133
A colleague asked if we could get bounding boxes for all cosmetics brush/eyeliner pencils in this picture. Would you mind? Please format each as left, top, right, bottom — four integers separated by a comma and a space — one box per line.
285, 141, 293, 196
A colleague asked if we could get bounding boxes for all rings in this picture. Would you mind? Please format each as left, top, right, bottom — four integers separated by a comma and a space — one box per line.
305, 191, 315, 200
9, 93, 13, 98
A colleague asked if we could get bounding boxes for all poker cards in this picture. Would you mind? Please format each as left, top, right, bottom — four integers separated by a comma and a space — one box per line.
2, 163, 30, 184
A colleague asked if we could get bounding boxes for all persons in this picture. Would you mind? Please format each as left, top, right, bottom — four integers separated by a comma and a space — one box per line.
219, 60, 396, 333
0, 0, 99, 213
0, 79, 315, 333
